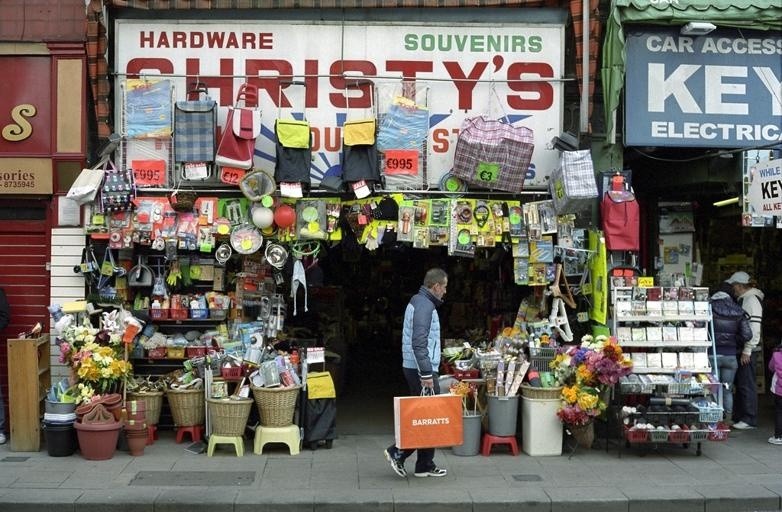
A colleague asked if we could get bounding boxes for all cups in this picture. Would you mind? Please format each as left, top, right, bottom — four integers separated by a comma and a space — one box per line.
211, 381, 226, 398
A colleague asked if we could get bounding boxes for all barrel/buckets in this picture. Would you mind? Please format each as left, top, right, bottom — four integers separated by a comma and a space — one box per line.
484, 393, 520, 437
452, 409, 483, 458
41, 399, 78, 456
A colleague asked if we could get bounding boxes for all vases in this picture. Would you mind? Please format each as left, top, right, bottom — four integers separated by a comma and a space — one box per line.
572, 421, 597, 451
42, 397, 151, 460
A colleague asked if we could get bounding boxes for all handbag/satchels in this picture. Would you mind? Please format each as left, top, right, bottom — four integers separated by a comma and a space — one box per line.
65, 169, 105, 206
549, 150, 599, 217
99, 169, 135, 217
451, 116, 534, 194
393, 386, 464, 450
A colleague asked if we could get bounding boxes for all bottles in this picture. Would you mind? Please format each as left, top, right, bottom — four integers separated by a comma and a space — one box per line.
275, 350, 302, 386
46, 303, 65, 323
526, 326, 557, 357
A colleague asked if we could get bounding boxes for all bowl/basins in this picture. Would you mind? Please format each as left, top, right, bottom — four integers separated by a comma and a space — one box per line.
231, 224, 265, 256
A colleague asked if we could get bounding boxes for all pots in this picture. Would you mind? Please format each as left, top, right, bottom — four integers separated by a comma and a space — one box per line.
264, 241, 289, 271
215, 243, 232, 266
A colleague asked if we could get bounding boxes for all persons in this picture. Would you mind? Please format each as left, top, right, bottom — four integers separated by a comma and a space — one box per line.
709, 282, 753, 427
768, 343, 782, 445
0, 287, 10, 445
384, 268, 447, 479
723, 270, 765, 430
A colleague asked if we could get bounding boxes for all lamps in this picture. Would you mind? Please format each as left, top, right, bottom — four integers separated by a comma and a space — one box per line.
550, 127, 581, 155
680, 20, 718, 36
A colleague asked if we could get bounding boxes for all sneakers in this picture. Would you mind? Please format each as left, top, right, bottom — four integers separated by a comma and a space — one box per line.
731, 421, 759, 430
384, 449, 407, 478
768, 436, 781, 445
414, 467, 447, 478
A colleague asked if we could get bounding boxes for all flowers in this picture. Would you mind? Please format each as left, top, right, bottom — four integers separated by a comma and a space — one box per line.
546, 329, 634, 429
56, 307, 135, 405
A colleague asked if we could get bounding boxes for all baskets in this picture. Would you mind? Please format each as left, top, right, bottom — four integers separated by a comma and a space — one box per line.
250, 383, 303, 427
208, 395, 254, 436
163, 380, 205, 427
619, 374, 720, 396
128, 390, 164, 424
692, 401, 723, 422
521, 382, 563, 399
527, 344, 559, 372
624, 422, 731, 443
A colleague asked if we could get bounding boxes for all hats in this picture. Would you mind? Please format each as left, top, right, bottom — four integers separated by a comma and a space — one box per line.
724, 272, 751, 285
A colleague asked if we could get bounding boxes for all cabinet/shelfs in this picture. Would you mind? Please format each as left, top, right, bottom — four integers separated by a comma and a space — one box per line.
606, 286, 730, 456
127, 318, 226, 361
7, 330, 51, 454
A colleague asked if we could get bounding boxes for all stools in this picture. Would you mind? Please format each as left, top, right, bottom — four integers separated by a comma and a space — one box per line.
174, 422, 206, 445
479, 431, 520, 458
204, 430, 247, 457
250, 423, 304, 457
